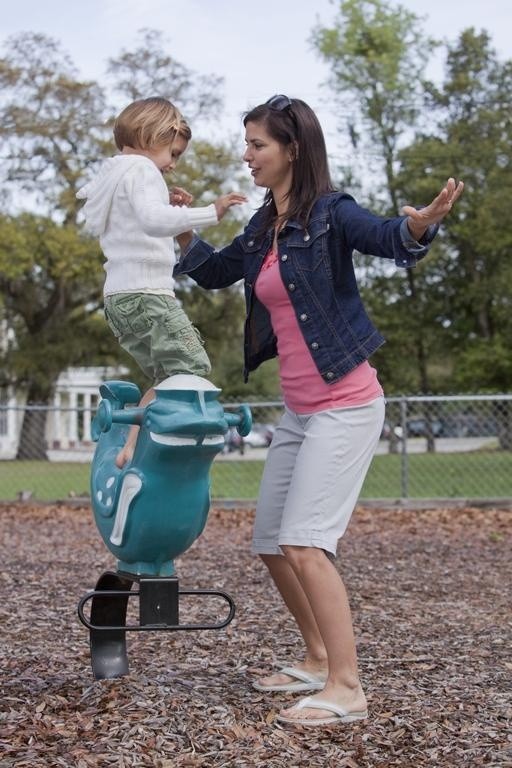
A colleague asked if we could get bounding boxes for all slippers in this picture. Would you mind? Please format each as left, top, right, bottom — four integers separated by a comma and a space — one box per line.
274, 695, 370, 726
252, 664, 326, 694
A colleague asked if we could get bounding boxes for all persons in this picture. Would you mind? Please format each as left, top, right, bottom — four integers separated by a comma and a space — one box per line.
73, 98, 247, 469
168, 93, 465, 726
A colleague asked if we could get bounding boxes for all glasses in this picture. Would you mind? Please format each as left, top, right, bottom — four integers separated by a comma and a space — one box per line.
267, 94, 299, 130
